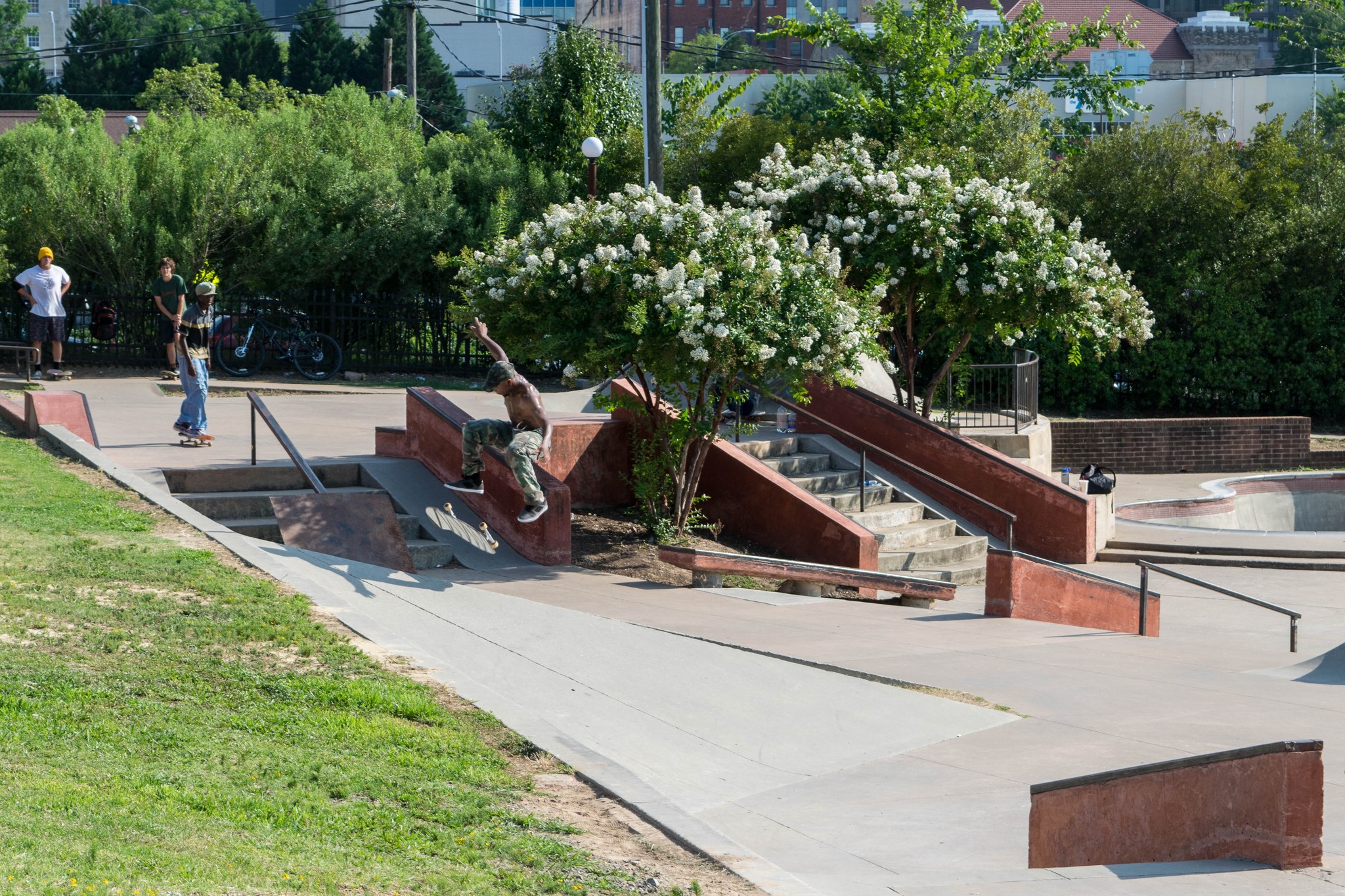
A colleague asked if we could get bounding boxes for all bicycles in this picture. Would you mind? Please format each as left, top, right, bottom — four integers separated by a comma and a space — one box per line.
208, 287, 344, 381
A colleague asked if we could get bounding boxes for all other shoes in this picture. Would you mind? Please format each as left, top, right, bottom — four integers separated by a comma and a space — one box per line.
173, 422, 192, 435
188, 428, 207, 437
167, 367, 180, 373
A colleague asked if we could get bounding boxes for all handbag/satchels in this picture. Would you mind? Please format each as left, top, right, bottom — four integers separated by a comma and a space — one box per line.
1086, 466, 1116, 494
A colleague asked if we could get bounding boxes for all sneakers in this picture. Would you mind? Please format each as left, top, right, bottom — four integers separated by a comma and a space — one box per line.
517, 496, 548, 523
444, 473, 484, 494
51, 375, 68, 380
33, 370, 43, 379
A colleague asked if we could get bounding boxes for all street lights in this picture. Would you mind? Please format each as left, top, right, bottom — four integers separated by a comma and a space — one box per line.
387, 84, 417, 128
495, 20, 502, 82
581, 136, 603, 201
48, 10, 57, 75
715, 29, 755, 71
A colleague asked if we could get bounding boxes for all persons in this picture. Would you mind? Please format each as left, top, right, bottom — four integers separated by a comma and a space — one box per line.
11, 247, 71, 380
172, 281, 218, 437
444, 317, 554, 523
152, 256, 188, 373
708, 370, 757, 425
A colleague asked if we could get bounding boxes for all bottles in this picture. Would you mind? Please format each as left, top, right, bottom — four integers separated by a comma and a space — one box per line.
858, 480, 877, 487
787, 411, 797, 432
776, 405, 787, 433
1061, 467, 1071, 486
417, 376, 425, 381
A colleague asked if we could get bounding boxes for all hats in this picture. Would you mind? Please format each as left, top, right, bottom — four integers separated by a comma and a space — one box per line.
481, 361, 515, 392
1080, 464, 1095, 480
38, 247, 53, 261
195, 282, 218, 296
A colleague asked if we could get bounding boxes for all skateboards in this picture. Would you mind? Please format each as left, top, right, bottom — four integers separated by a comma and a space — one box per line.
160, 371, 180, 381
178, 432, 216, 447
47, 369, 72, 380
426, 502, 499, 554
718, 410, 767, 422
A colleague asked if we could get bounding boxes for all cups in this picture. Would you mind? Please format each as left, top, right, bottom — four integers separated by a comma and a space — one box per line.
1078, 479, 1089, 494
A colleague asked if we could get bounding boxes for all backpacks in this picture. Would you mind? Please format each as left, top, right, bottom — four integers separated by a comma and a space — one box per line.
90, 300, 116, 341
